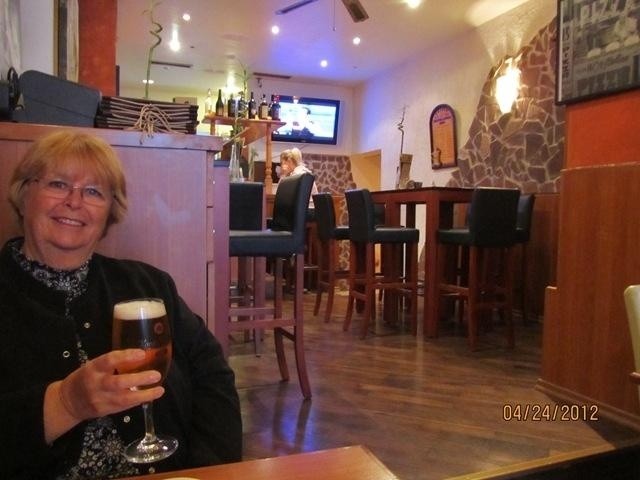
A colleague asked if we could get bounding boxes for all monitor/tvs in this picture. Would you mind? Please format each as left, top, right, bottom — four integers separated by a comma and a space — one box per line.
271, 95, 340, 145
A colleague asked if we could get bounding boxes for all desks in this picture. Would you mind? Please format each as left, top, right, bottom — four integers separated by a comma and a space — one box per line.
94, 444, 400, 480
356, 187, 496, 340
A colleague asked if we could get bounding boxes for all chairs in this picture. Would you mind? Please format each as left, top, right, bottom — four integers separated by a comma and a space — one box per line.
343, 189, 419, 339
437, 188, 521, 352
373, 201, 405, 312
230, 171, 314, 399
486, 194, 535, 331
312, 194, 375, 323
623, 285, 640, 384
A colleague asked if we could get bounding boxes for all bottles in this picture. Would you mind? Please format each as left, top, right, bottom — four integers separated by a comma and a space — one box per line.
228, 94, 236, 117
238, 91, 245, 118
216, 89, 224, 116
258, 93, 280, 120
205, 89, 212, 114
230, 145, 237, 182
112, 298, 179, 464
249, 92, 256, 119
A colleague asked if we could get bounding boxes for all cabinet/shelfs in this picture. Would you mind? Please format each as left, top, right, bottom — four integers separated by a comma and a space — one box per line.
0, 122, 224, 336
202, 113, 286, 217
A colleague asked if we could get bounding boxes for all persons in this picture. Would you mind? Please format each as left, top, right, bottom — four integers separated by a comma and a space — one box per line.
1, 131, 242, 480
290, 107, 316, 137
276, 146, 319, 223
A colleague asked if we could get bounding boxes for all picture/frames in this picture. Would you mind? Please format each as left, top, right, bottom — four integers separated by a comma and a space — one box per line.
430, 104, 457, 169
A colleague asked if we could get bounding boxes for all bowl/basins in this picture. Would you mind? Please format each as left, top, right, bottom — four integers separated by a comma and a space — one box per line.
413, 182, 423, 187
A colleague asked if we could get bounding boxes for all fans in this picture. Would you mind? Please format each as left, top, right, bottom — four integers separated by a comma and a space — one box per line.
276, 0, 370, 24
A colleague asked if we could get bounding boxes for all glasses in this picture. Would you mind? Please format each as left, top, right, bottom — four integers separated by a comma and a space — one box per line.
26, 177, 114, 208
